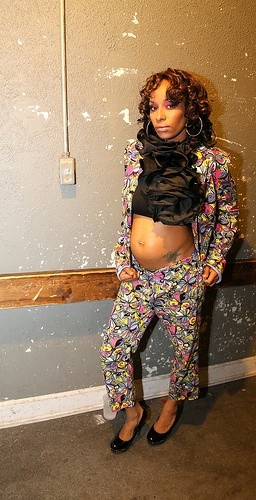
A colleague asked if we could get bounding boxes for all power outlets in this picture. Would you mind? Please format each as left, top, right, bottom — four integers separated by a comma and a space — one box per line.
60, 158, 75, 185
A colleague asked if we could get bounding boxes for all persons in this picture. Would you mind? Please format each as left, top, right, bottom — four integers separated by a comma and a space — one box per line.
98, 66, 239, 452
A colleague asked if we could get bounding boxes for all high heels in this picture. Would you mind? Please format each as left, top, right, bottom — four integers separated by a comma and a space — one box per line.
110, 409, 147, 455
146, 403, 183, 445
